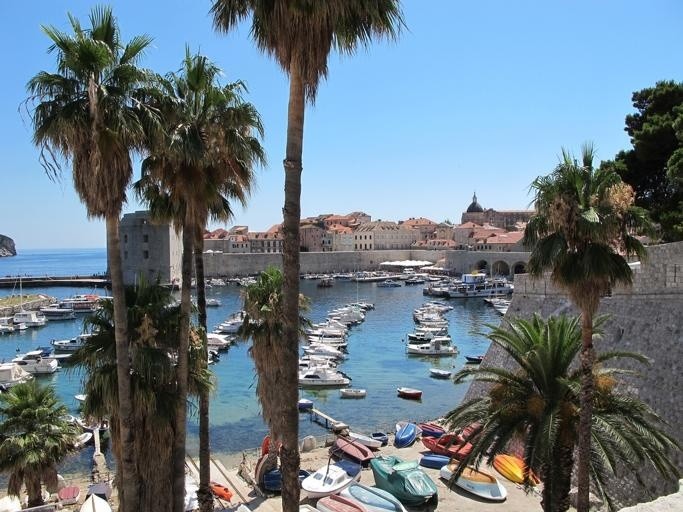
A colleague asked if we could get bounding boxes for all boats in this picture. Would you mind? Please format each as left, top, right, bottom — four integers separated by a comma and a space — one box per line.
428, 368, 452, 379
189, 275, 262, 366
397, 265, 514, 299
0, 278, 113, 333
296, 300, 374, 386
483, 297, 512, 316
0, 329, 115, 390
405, 300, 459, 356
299, 418, 543, 512
339, 388, 367, 398
463, 355, 484, 362
0, 471, 112, 512
396, 386, 423, 398
0, 394, 110, 467
299, 267, 402, 288
297, 397, 314, 412
